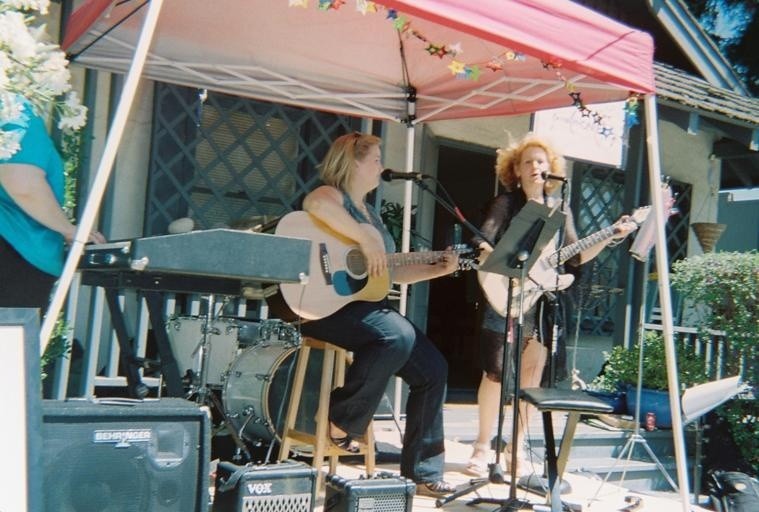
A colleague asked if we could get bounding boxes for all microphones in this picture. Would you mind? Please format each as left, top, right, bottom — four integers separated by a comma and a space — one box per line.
380, 166, 428, 185
540, 170, 567, 186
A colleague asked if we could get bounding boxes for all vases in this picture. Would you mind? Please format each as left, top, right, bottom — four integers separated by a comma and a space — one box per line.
628, 383, 683, 430
708, 287, 738, 313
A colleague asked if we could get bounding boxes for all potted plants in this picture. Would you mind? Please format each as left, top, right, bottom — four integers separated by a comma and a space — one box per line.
570, 349, 627, 414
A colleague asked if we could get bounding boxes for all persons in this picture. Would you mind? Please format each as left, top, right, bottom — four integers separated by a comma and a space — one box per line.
465, 132, 638, 480
1, 86, 108, 324
302, 131, 460, 494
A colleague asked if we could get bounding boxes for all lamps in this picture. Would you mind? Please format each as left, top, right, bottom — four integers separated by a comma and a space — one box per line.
686, 111, 699, 136
748, 127, 759, 152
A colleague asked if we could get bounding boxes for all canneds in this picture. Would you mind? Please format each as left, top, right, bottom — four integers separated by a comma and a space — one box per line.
646, 413, 656, 431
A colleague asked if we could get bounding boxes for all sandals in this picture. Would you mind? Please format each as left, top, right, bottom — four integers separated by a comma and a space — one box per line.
504, 443, 532, 476
331, 434, 360, 453
414, 478, 456, 494
467, 440, 491, 476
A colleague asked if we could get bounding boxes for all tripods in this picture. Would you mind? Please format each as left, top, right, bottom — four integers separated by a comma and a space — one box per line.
183, 290, 253, 463
588, 261, 682, 512
420, 188, 548, 506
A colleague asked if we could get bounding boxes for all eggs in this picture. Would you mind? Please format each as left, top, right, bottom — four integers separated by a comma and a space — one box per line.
168, 218, 194, 233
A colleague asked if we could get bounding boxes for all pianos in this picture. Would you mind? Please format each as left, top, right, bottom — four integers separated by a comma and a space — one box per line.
64, 227, 311, 294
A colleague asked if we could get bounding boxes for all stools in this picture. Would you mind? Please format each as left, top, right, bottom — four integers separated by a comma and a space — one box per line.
519, 388, 615, 512
275, 336, 376, 512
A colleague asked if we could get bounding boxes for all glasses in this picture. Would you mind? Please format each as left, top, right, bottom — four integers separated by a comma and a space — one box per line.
354, 132, 361, 147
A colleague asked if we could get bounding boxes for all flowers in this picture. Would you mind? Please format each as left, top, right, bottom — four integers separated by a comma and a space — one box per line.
596, 332, 710, 399
669, 250, 759, 394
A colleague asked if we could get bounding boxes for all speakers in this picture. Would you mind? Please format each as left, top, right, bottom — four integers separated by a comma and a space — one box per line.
212, 459, 318, 512
41, 395, 212, 512
323, 470, 417, 512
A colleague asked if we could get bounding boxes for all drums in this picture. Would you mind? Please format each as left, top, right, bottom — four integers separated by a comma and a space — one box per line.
164, 313, 239, 389
221, 342, 355, 459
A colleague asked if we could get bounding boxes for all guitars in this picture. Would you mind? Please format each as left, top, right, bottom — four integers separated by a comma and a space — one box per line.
477, 205, 655, 319
262, 210, 485, 327
